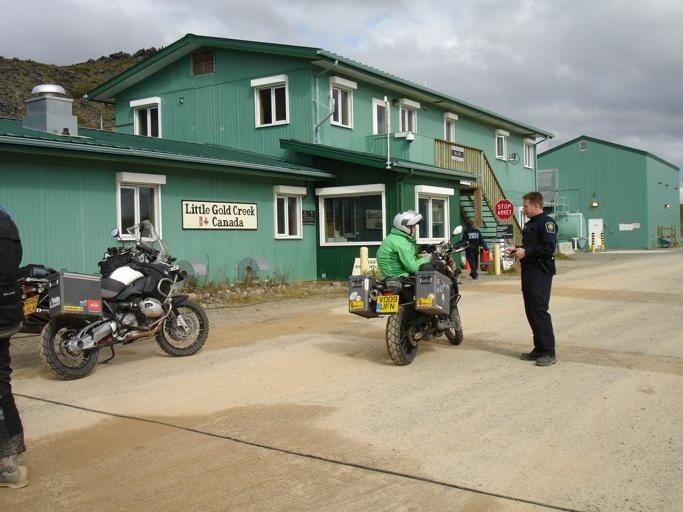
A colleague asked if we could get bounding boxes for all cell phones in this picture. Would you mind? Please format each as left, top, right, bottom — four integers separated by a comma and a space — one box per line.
508, 249, 516, 256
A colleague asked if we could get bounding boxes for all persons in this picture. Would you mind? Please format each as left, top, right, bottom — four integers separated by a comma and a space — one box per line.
375, 209, 461, 283
501, 192, 558, 366
0, 204, 31, 490
461, 219, 491, 282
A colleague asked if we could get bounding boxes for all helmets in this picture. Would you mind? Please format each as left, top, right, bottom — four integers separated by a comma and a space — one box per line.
392, 209, 425, 237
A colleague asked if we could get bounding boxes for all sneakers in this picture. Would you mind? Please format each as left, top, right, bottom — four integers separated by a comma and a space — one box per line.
0, 453, 29, 490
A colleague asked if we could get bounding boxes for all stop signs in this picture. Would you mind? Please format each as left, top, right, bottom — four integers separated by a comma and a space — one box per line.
495, 199, 514, 220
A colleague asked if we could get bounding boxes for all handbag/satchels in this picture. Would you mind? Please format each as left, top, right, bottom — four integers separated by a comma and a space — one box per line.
0, 285, 24, 338
537, 257, 556, 277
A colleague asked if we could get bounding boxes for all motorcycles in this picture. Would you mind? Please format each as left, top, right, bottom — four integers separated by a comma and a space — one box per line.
16, 219, 210, 382
347, 224, 470, 367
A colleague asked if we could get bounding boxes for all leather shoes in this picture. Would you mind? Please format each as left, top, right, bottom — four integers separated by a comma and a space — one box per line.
535, 354, 558, 367
520, 348, 540, 361
